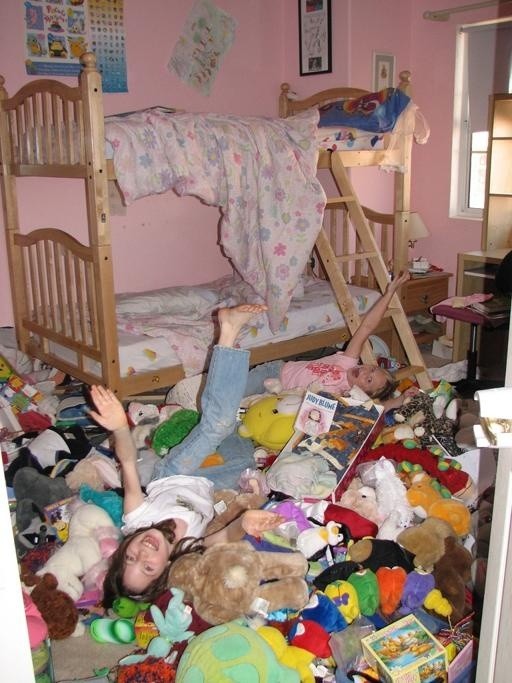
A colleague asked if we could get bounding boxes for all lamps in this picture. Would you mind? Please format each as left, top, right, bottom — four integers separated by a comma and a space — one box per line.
404, 211, 432, 250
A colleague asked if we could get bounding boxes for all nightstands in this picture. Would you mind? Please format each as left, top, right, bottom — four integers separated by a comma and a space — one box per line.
351, 269, 454, 343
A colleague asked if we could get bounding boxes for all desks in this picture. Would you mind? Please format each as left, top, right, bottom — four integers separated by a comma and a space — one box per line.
454, 247, 511, 372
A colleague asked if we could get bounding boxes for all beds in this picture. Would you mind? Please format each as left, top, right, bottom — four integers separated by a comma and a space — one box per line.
1, 52, 436, 188
3, 199, 414, 393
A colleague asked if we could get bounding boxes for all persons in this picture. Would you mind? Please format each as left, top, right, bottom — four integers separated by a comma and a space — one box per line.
87, 303, 287, 603
244, 270, 416, 399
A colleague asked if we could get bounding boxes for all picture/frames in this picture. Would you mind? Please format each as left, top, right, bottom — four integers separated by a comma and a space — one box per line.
372, 52, 396, 91
297, 0, 332, 77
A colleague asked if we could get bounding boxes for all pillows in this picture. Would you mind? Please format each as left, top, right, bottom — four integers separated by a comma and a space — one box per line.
315, 86, 411, 137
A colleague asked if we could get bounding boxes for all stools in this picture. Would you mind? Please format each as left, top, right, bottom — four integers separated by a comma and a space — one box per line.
433, 302, 496, 400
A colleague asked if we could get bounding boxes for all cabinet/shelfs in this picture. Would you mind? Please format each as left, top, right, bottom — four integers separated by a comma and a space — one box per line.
481, 92, 512, 248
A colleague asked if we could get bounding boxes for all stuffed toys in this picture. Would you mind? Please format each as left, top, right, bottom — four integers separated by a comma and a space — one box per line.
1, 383, 120, 640
119, 545, 482, 678
213, 387, 499, 548
